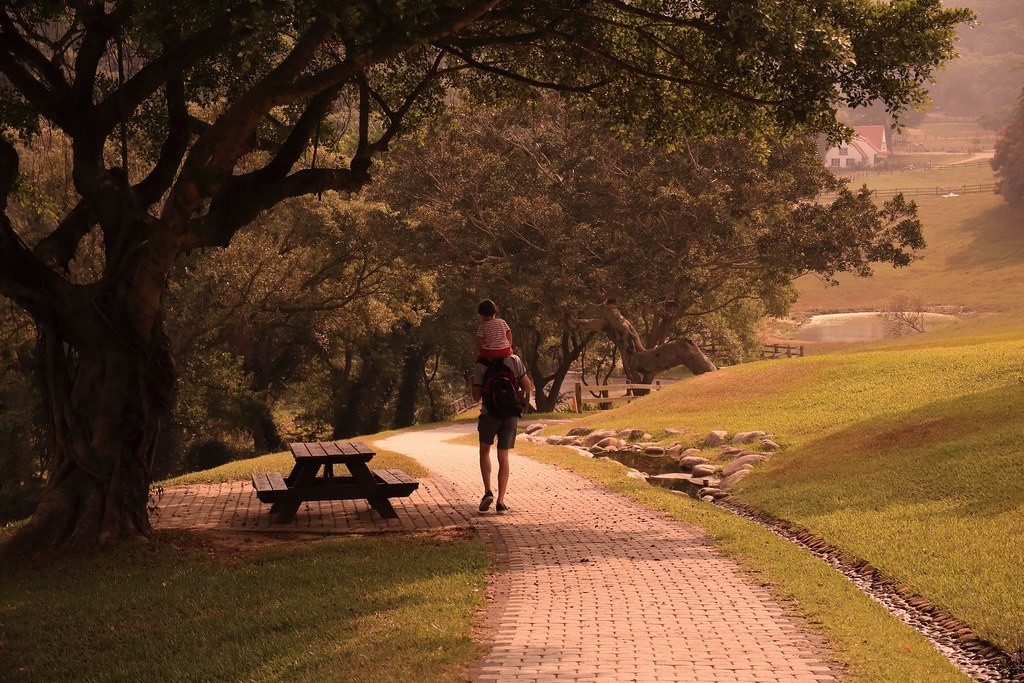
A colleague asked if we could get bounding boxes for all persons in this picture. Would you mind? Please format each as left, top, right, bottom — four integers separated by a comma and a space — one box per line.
471, 356, 531, 515
477, 300, 513, 356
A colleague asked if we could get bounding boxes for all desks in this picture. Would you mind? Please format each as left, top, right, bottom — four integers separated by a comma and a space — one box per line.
269, 442, 401, 524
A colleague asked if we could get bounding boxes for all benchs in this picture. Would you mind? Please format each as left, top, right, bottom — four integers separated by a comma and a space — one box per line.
251, 472, 287, 504
370, 468, 419, 498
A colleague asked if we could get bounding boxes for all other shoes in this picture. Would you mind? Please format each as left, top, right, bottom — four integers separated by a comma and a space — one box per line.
479, 490, 494, 511
496, 503, 508, 514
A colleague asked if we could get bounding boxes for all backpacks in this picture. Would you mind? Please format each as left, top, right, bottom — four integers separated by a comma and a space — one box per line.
478, 356, 524, 418
